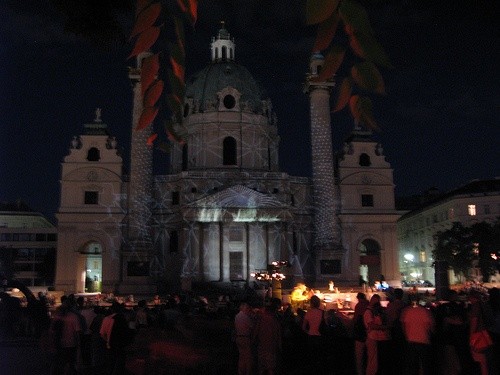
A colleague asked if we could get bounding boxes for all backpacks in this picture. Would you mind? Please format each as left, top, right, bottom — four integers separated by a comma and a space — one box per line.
353, 308, 375, 342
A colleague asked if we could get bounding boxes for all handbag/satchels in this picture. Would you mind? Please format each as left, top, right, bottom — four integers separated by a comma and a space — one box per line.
472, 330, 494, 352
319, 310, 329, 337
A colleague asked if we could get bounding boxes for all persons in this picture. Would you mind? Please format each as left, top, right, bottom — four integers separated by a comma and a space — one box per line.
0, 289, 209, 375
232, 282, 500, 375
